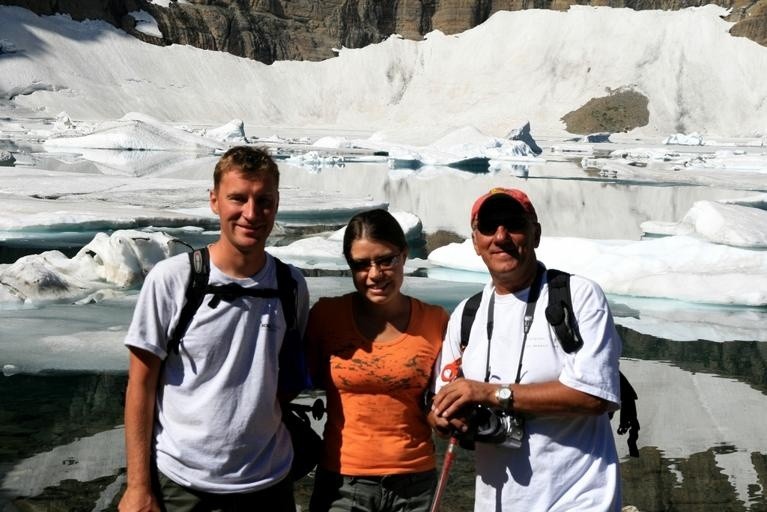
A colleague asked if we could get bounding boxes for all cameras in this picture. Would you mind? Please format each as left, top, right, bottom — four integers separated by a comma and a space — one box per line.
455, 402, 525, 450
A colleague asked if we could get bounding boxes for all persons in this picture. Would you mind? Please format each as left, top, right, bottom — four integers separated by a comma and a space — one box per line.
426, 188, 622, 512
300, 208, 450, 512
117, 145, 309, 512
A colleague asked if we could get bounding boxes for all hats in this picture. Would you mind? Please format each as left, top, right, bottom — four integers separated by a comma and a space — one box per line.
470, 185, 538, 221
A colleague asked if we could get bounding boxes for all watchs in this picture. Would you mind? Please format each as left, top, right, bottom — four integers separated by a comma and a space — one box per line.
495, 384, 513, 408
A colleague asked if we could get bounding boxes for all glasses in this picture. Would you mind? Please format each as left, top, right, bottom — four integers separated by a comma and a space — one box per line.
349, 253, 403, 268
